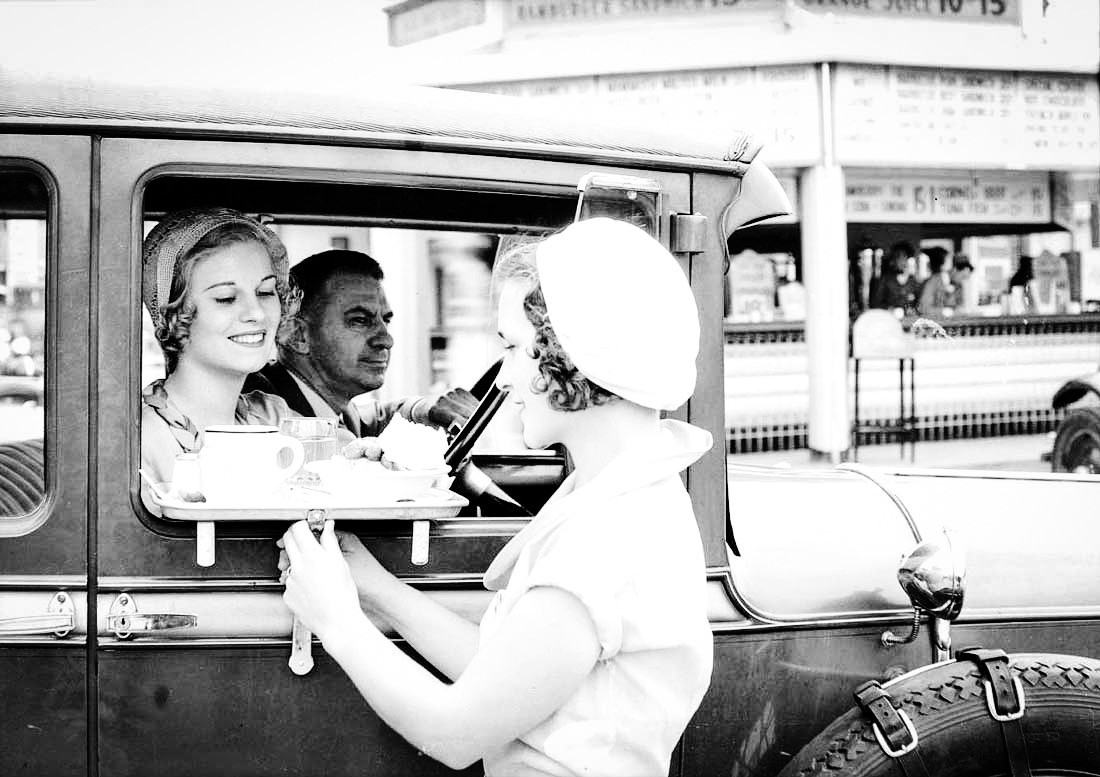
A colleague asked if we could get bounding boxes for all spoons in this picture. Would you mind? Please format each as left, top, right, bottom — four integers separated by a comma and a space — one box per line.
138, 468, 183, 503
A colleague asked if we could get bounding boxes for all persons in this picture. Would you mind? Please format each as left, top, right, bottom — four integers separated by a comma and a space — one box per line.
141, 206, 478, 518
276, 217, 716, 777
874, 245, 1037, 318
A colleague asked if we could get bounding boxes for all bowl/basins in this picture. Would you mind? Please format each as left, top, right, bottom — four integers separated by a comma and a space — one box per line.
307, 461, 452, 508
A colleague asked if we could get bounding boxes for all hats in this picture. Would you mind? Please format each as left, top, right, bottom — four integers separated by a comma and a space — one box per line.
143, 206, 291, 340
532, 215, 702, 413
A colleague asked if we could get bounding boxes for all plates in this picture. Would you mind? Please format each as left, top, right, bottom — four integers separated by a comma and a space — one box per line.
148, 478, 469, 521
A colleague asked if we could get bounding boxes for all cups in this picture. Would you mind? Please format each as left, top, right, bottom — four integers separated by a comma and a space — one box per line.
202, 425, 304, 510
280, 416, 340, 486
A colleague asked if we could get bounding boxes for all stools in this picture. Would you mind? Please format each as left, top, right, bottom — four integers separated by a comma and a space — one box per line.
851, 308, 917, 464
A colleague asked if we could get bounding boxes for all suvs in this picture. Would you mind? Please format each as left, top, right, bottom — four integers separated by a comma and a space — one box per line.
1, 97, 1099, 777
